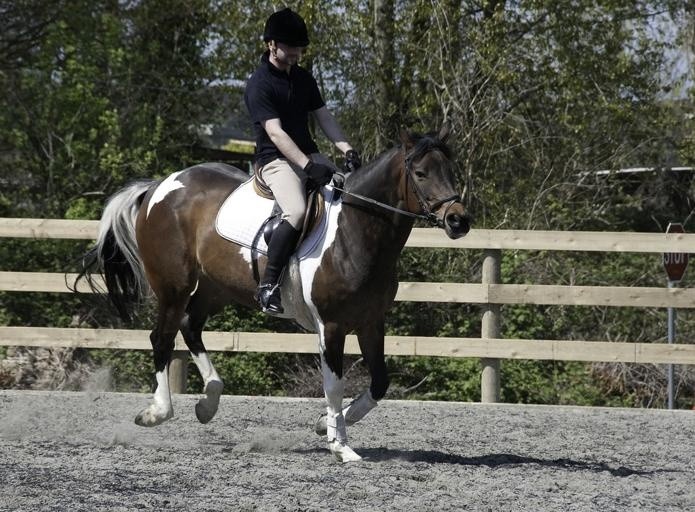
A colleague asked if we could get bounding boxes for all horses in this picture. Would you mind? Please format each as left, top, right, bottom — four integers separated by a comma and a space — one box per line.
63, 119, 474, 468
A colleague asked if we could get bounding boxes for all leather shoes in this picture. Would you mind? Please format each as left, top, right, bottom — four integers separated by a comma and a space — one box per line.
253, 280, 284, 313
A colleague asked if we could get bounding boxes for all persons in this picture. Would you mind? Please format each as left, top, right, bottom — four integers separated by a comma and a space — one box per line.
243, 9, 363, 315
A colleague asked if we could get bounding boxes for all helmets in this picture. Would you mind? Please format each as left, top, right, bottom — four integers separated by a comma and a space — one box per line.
264, 7, 310, 47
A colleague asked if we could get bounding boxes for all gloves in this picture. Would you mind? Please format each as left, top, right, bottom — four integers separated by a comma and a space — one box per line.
303, 161, 331, 185
343, 151, 362, 171
